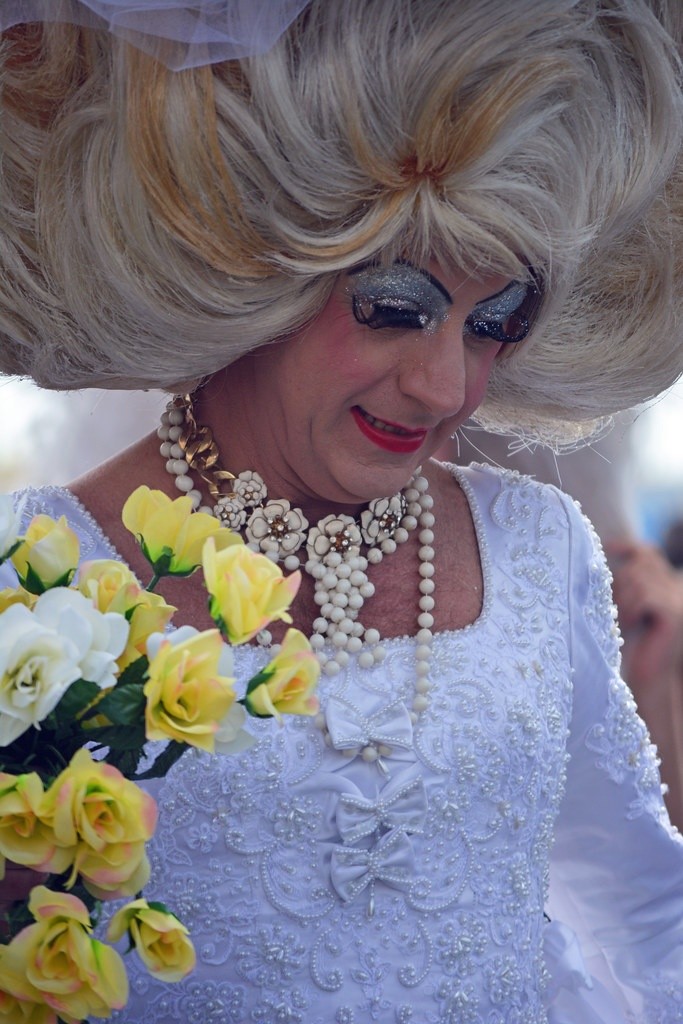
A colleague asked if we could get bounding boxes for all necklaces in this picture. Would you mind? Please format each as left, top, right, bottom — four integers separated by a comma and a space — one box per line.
159, 394, 435, 763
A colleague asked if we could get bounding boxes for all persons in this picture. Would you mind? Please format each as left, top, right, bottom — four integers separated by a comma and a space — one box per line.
437, 413, 683, 836
1, 0, 683, 1024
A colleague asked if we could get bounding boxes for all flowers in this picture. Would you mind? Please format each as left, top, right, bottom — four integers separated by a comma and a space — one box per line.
0, 484, 321, 1024
